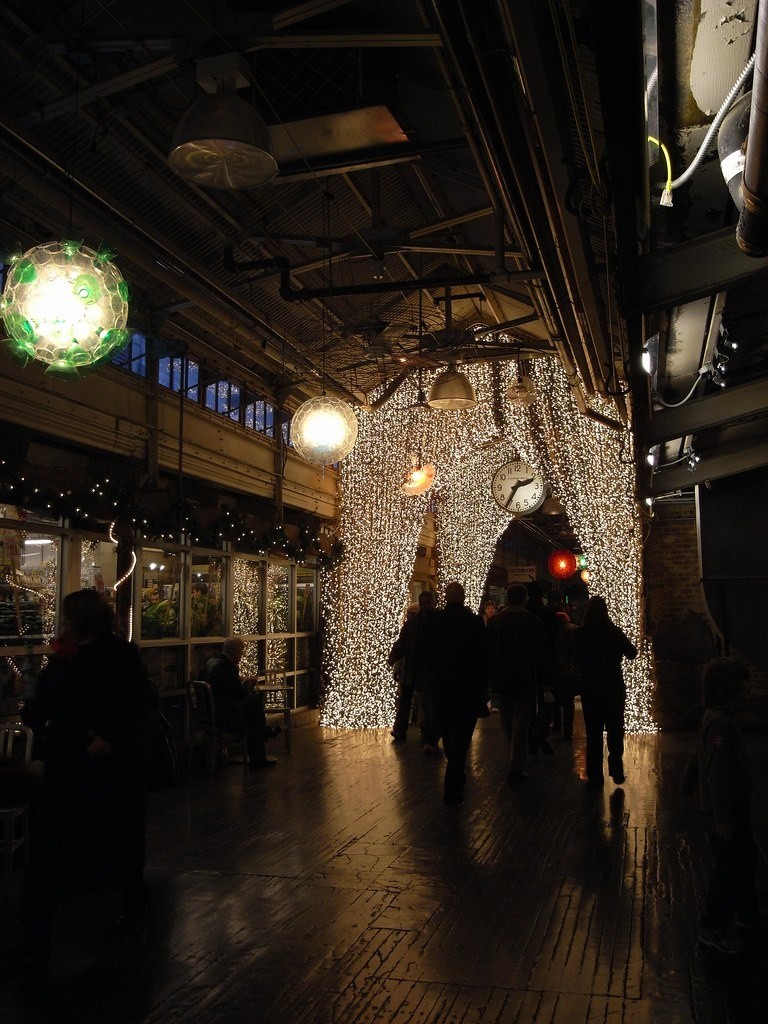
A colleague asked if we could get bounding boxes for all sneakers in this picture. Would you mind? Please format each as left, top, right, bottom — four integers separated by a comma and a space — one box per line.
696, 912, 764, 954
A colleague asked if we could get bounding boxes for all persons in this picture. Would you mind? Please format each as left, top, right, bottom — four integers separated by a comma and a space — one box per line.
0, 583, 157, 974
389, 583, 636, 809
205, 639, 281, 768
697, 654, 768, 954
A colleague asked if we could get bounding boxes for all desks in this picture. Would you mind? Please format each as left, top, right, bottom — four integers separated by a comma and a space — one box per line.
153, 689, 194, 782
250, 684, 295, 752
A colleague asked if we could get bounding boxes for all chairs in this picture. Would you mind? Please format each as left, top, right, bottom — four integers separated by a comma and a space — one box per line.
262, 669, 292, 749
184, 680, 249, 785
0, 724, 35, 899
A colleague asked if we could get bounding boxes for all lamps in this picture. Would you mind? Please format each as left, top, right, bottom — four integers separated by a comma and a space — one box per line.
0, 49, 132, 381
429, 286, 477, 411
287, 190, 359, 466
167, 50, 280, 191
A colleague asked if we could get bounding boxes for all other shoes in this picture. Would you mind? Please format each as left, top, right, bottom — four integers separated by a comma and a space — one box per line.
423, 743, 438, 754
443, 795, 464, 808
391, 730, 407, 740
251, 756, 277, 768
609, 767, 626, 785
506, 772, 528, 784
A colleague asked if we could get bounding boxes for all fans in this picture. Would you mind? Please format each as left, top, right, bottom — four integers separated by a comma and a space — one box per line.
283, 250, 475, 393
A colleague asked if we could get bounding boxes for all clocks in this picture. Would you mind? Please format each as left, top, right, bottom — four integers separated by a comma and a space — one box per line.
493, 462, 548, 516
